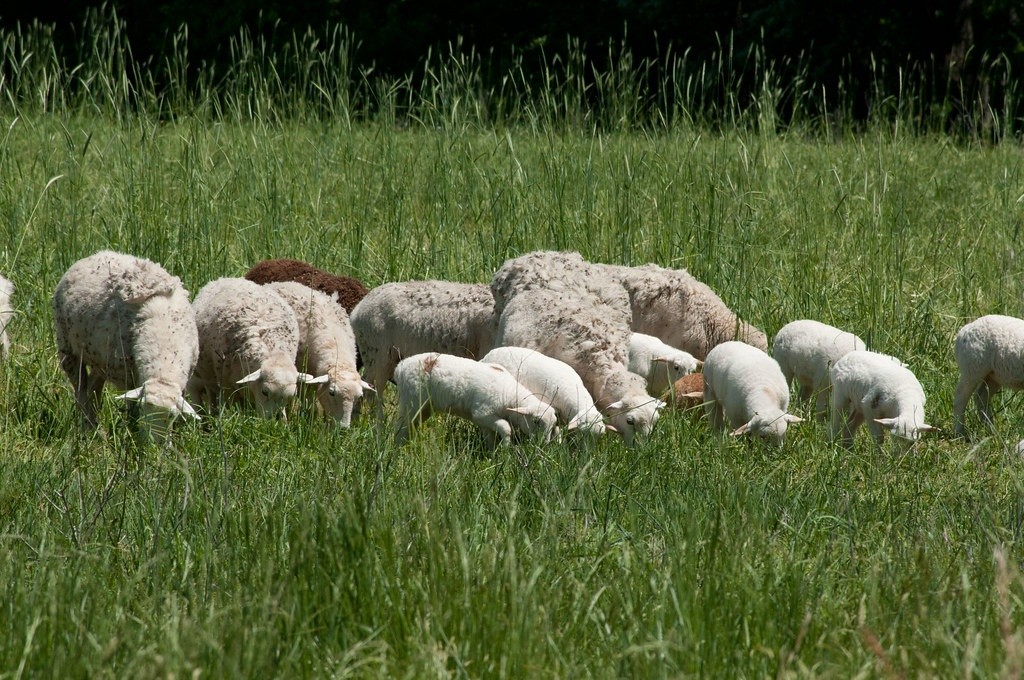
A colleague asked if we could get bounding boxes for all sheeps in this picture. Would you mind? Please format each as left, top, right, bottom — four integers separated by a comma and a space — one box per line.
49, 248, 943, 456
951, 314, 1024, 444
